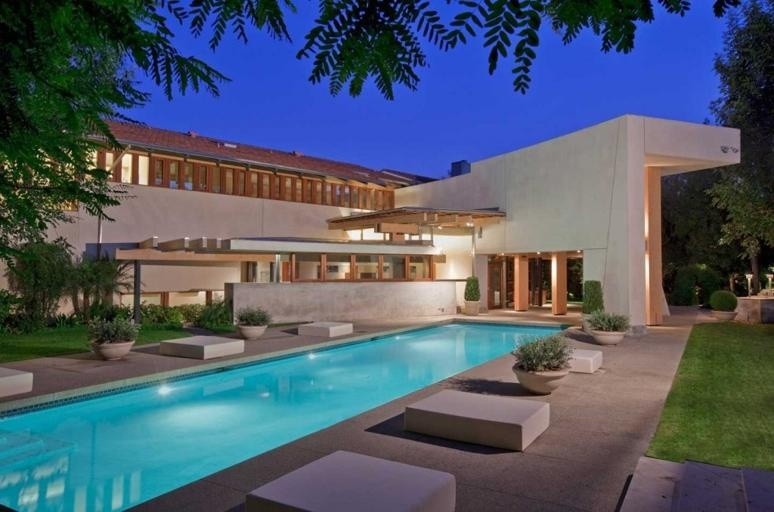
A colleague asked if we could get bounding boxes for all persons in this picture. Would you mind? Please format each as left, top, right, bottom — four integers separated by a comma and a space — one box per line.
695, 284, 704, 306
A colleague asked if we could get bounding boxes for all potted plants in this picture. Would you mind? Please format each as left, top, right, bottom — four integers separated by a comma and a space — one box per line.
582, 280, 605, 331
511, 334, 574, 394
709, 290, 739, 320
237, 306, 271, 340
87, 316, 143, 360
465, 277, 481, 315
588, 311, 632, 345
